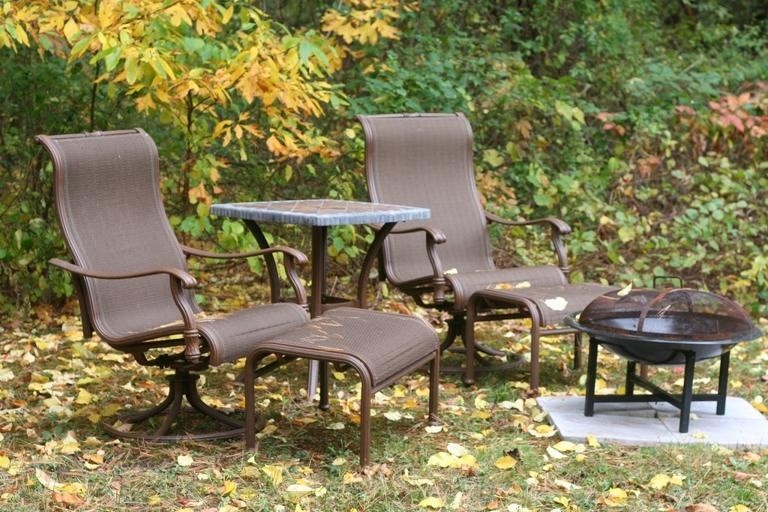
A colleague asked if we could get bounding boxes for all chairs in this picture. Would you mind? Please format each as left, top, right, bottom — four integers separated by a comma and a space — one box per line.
34, 128, 314, 443
353, 111, 573, 373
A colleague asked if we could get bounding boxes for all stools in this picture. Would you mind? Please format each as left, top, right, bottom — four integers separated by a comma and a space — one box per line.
464, 280, 625, 393
242, 307, 443, 466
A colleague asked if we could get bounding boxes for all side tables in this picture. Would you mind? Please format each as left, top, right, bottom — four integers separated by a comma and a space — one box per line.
209, 200, 429, 409
